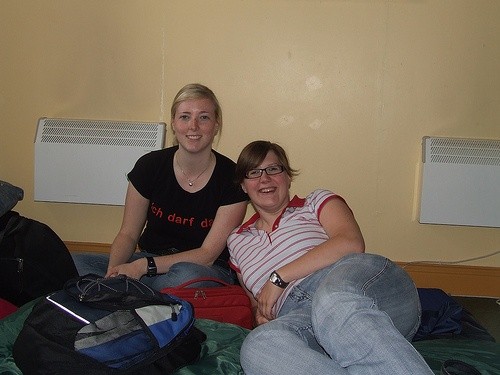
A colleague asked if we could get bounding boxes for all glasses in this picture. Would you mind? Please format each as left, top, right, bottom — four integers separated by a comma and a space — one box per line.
242, 164, 285, 179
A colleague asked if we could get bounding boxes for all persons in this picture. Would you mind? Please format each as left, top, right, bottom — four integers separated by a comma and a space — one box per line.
69, 82, 251, 295
225, 139, 436, 375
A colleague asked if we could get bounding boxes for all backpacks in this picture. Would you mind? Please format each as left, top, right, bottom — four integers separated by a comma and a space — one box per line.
12, 272, 207, 375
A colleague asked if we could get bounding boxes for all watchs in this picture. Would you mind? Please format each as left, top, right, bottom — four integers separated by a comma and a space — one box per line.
269, 271, 290, 289
143, 256, 157, 276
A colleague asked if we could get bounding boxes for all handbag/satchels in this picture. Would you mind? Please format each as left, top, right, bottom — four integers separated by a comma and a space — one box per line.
0, 210, 80, 309
160, 277, 252, 330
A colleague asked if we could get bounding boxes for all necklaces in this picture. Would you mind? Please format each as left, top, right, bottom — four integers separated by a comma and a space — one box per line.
175, 149, 212, 186
